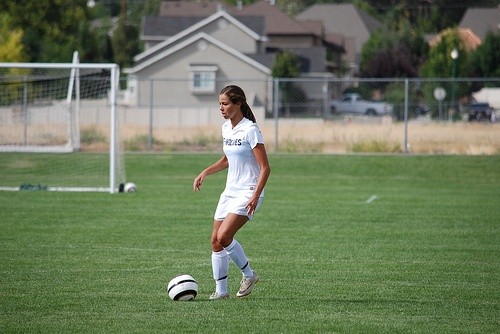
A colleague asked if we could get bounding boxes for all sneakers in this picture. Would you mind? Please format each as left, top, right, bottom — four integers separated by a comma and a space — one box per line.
236, 270, 258, 298
208, 291, 229, 301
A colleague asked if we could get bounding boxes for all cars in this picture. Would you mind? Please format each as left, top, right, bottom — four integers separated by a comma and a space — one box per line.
331, 93, 394, 116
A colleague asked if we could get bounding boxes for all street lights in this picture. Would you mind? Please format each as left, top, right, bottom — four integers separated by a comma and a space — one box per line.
451, 50, 459, 122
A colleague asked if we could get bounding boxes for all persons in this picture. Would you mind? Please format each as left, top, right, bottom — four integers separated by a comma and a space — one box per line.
193, 85, 270, 302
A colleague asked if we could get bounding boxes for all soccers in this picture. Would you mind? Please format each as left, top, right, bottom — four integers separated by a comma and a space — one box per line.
168, 275, 198, 301
123, 182, 137, 192
